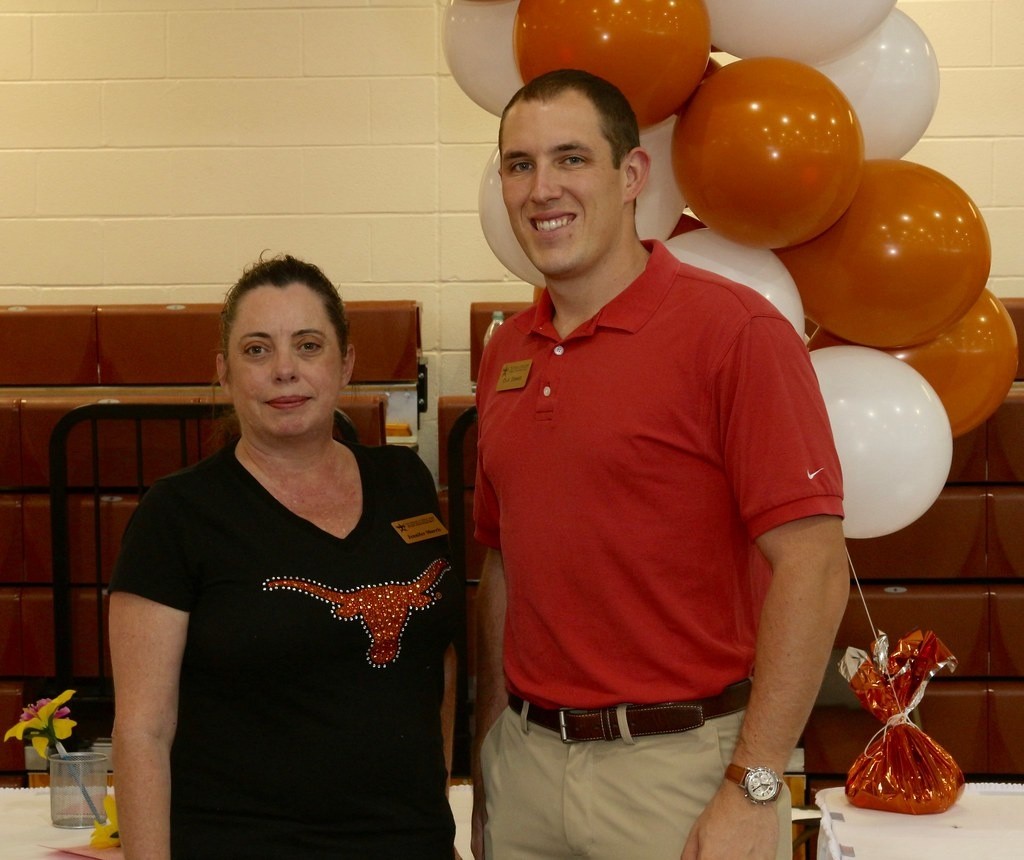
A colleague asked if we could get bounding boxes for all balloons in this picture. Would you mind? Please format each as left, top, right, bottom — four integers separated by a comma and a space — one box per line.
512, 0, 711, 128
771, 160, 992, 348
660, 228, 804, 343
804, 287, 1019, 438
479, 141, 547, 289
806, 345, 953, 539
671, 56, 865, 249
814, 6, 940, 159
635, 56, 722, 241
444, 0, 526, 118
705, 0, 895, 62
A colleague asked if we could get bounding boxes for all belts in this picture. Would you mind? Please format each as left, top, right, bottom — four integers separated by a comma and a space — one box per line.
504, 676, 754, 745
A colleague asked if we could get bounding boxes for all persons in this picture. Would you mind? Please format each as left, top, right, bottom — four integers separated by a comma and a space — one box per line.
107, 253, 461, 860
468, 67, 850, 859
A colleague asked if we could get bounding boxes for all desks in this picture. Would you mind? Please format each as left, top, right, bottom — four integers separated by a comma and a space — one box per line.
0, 785, 115, 860
814, 780, 1024, 860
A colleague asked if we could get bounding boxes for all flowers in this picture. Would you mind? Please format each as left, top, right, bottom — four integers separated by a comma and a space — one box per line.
3, 690, 77, 760
88, 794, 120, 847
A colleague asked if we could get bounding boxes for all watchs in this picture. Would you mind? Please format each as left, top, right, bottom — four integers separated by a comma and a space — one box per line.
724, 764, 783, 805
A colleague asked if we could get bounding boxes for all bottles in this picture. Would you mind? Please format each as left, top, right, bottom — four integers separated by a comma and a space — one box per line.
484, 310, 504, 347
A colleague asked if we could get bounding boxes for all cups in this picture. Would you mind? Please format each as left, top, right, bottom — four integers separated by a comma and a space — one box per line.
48, 752, 108, 828
